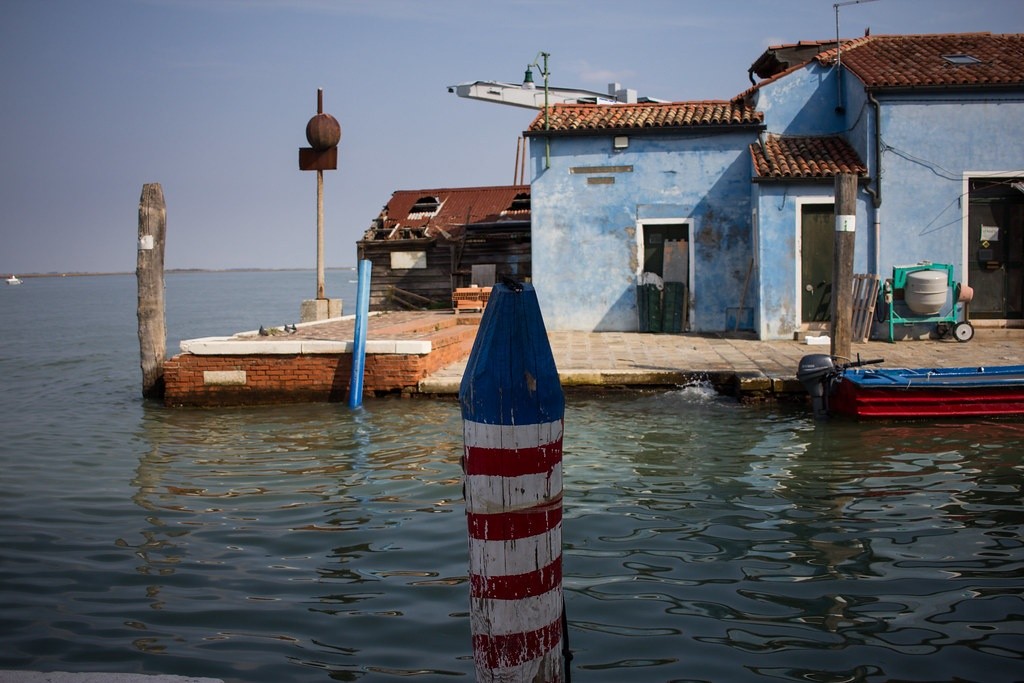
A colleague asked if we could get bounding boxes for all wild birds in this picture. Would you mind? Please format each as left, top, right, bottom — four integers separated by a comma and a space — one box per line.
259, 325, 268, 336
284, 323, 297, 334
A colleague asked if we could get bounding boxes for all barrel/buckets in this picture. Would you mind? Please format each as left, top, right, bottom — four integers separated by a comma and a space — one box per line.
956, 281, 974, 303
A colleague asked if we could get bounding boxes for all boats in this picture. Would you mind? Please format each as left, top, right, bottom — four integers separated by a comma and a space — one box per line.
5, 275, 23, 286
796, 353, 1024, 418
